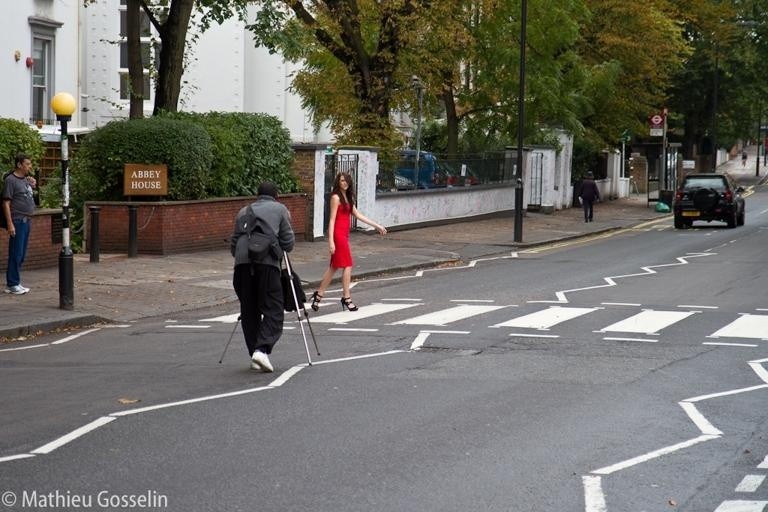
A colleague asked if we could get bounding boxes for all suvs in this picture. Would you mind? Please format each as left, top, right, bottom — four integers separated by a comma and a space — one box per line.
671, 171, 748, 233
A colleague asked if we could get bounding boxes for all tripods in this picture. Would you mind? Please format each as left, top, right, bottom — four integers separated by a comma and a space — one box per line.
219, 248, 320, 366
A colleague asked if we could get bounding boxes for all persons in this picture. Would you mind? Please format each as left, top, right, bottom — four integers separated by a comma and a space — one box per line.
230, 181, 295, 374
308, 173, 387, 312
577, 171, 600, 224
0, 154, 40, 295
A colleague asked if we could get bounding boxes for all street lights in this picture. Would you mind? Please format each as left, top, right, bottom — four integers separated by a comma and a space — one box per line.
48, 90, 80, 310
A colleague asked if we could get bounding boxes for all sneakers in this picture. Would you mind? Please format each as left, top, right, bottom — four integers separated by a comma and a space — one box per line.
251, 351, 273, 372
4, 284, 30, 295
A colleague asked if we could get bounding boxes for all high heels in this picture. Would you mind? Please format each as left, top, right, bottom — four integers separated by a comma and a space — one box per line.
341, 297, 358, 311
309, 291, 322, 311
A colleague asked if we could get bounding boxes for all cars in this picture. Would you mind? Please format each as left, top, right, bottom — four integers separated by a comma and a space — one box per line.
365, 148, 480, 195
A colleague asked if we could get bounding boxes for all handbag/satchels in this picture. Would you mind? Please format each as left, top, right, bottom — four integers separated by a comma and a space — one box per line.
248, 232, 273, 259
282, 269, 306, 311
